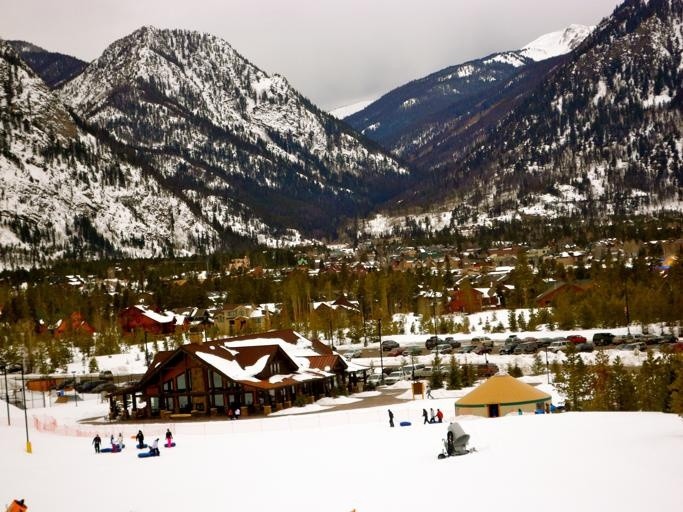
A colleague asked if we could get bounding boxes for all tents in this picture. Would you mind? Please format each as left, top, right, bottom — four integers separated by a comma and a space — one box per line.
454, 368, 552, 418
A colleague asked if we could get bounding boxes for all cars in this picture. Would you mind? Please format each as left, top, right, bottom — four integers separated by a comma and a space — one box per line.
343, 348, 362, 359
48, 370, 142, 392
352, 363, 499, 387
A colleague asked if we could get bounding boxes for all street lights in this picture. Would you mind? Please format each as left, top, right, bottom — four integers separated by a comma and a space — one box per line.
377, 317, 387, 386
144, 329, 150, 369
326, 318, 335, 350
431, 300, 440, 359
7, 360, 30, 454
0, 360, 11, 425
71, 370, 78, 406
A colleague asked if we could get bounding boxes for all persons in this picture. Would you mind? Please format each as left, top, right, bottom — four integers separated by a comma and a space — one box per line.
108, 435, 118, 453
429, 408, 436, 423
150, 438, 160, 457
225, 407, 240, 420
387, 408, 394, 427
91, 434, 101, 454
134, 429, 145, 449
147, 446, 154, 453
164, 428, 173, 448
421, 408, 430, 426
425, 385, 434, 400
435, 408, 443, 424
116, 433, 124, 449
